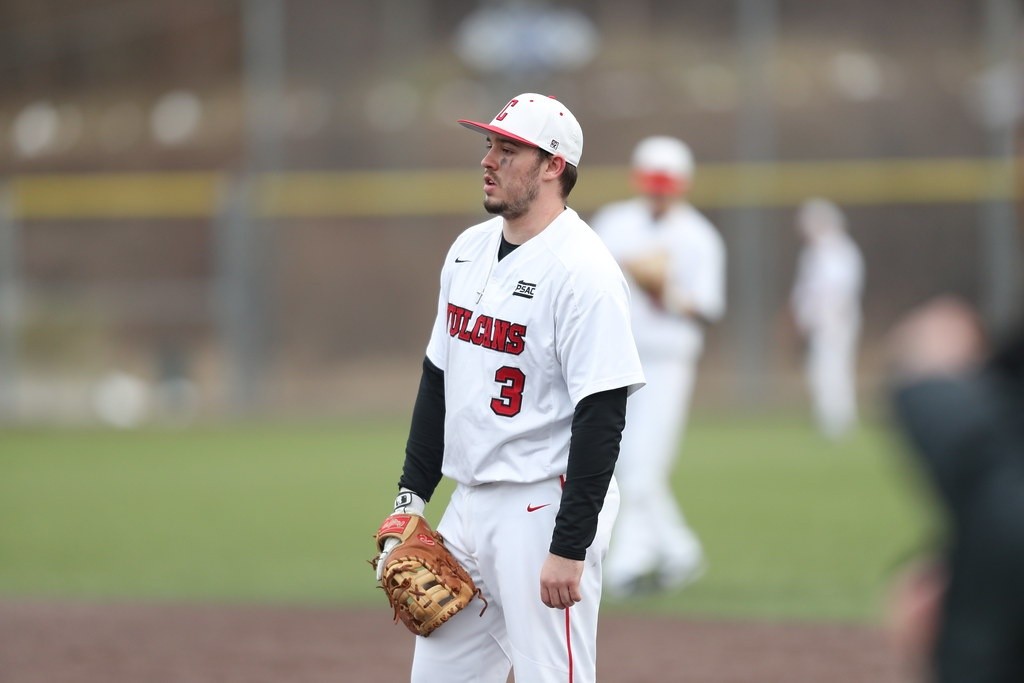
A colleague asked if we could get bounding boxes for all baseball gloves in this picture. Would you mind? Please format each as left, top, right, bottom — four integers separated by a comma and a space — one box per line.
367, 514, 489, 638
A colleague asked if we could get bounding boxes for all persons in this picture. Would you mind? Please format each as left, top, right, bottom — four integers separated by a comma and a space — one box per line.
874, 282, 1024, 683
788, 194, 870, 440
377, 89, 648, 683
589, 133, 731, 596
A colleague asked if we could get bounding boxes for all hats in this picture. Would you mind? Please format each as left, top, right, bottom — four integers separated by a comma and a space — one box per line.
458, 94, 583, 166
626, 135, 695, 196
